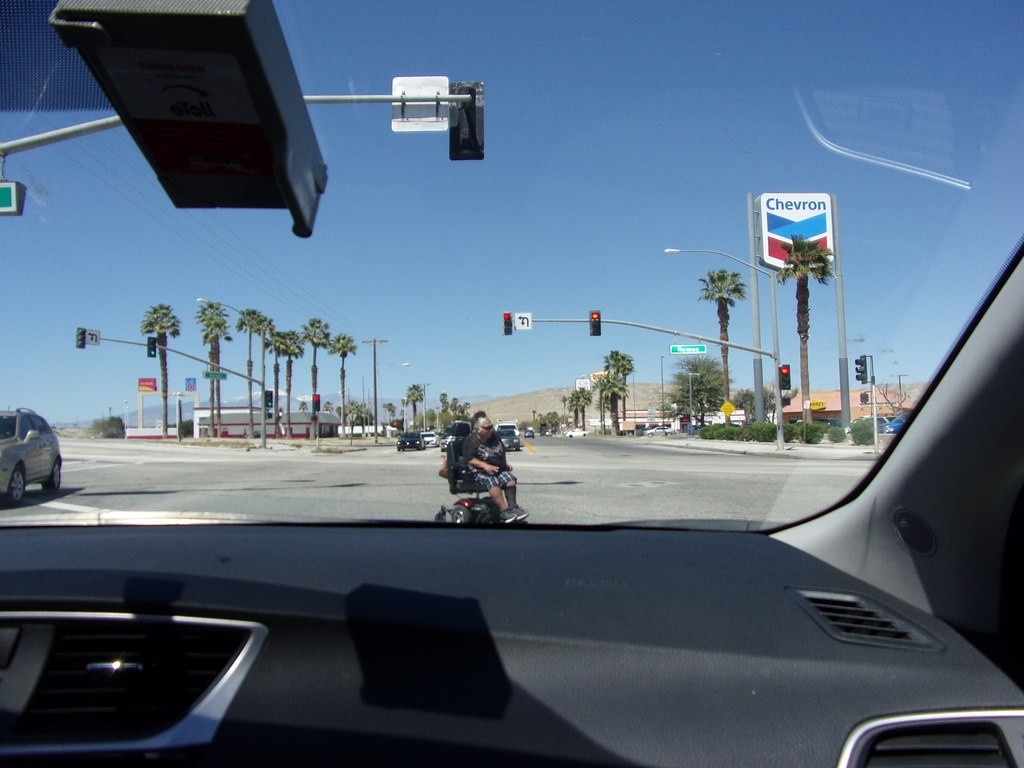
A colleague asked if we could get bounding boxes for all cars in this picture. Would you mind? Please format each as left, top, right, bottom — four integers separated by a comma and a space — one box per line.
244, 429, 261, 438
524, 426, 553, 438
643, 426, 676, 437
565, 429, 587, 438
494, 420, 521, 451
396, 420, 472, 452
845, 415, 896, 433
876, 411, 910, 435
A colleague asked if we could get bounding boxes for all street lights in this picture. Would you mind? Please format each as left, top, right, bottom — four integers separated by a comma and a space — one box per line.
664, 248, 784, 452
560, 396, 567, 425
633, 370, 638, 436
532, 410, 536, 421
196, 298, 254, 439
689, 372, 700, 437
660, 355, 665, 437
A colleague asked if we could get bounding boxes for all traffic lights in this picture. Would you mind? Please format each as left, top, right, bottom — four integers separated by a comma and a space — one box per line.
76, 327, 86, 349
312, 394, 321, 411
589, 309, 601, 336
504, 312, 512, 335
264, 390, 273, 408
855, 355, 867, 384
778, 364, 790, 390
147, 335, 157, 357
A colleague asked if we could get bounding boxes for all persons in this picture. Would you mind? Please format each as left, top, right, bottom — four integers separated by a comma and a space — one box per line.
463, 416, 529, 523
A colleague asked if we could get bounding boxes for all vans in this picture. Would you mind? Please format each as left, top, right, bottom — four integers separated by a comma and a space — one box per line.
812, 417, 841, 427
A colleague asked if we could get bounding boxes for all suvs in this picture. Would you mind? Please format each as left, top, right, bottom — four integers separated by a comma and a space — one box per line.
0, 408, 63, 506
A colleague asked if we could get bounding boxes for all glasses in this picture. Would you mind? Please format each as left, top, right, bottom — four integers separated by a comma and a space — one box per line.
482, 425, 493, 431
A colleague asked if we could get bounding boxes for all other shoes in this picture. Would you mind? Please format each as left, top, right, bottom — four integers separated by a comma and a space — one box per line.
499, 509, 518, 523
508, 505, 528, 520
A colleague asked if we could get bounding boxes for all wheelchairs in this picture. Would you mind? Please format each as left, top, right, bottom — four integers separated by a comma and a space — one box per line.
434, 439, 530, 526
